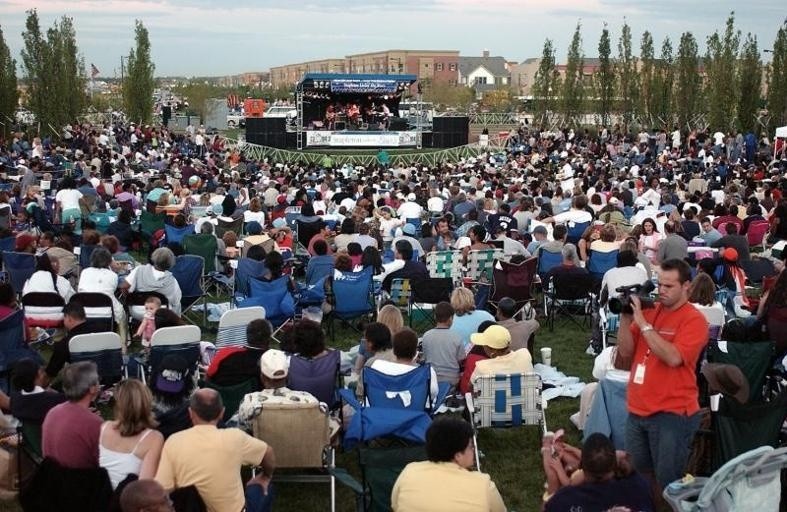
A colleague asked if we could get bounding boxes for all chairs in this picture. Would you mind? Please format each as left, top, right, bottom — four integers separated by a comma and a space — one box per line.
0, 184, 770, 510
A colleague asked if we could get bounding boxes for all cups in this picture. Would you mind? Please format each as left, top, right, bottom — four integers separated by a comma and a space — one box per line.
539, 346, 553, 365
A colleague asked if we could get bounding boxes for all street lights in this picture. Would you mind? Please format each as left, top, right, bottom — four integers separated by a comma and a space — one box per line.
305, 80, 411, 101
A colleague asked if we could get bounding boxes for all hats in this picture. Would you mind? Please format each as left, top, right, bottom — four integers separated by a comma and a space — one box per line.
529, 227, 547, 234
700, 362, 750, 403
723, 247, 738, 261
260, 348, 289, 378
403, 224, 416, 235
470, 325, 510, 348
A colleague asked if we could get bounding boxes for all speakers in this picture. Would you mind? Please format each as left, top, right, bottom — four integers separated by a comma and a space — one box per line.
246, 117, 306, 150
422, 117, 469, 148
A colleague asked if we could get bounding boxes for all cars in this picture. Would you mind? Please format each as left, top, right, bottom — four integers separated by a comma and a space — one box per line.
151, 98, 298, 130
15, 106, 128, 129
396, 100, 480, 123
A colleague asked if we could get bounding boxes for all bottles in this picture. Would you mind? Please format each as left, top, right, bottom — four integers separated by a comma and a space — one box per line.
34, 326, 56, 346
70, 214, 76, 224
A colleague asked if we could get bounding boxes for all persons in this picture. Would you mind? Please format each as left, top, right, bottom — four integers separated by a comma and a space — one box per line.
0, 103, 787, 512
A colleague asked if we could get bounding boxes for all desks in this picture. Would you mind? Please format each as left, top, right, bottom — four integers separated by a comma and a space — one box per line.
333, 120, 347, 130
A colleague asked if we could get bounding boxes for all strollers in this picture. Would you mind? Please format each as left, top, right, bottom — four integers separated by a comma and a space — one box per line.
661, 443, 786, 512
